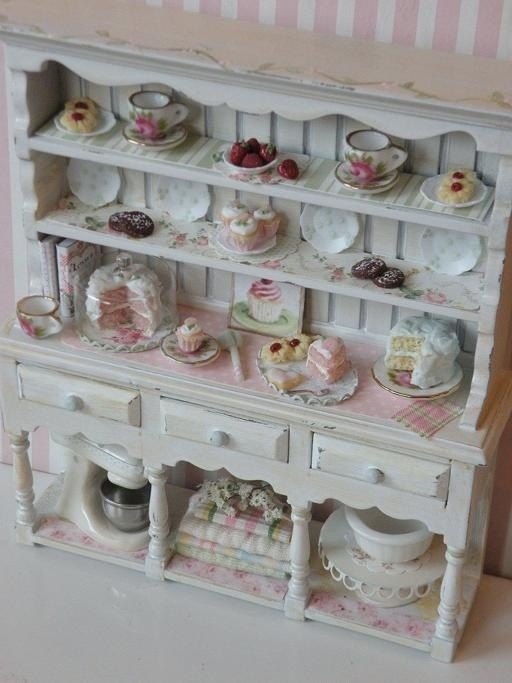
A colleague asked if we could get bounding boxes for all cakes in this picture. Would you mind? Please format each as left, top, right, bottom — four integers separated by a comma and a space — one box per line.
383, 316, 460, 391
246, 278, 286, 324
84, 262, 164, 340
218, 202, 282, 252
261, 333, 347, 391
174, 316, 205, 354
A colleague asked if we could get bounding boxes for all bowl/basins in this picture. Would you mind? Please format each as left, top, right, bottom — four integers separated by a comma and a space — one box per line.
98, 475, 152, 533
344, 506, 437, 565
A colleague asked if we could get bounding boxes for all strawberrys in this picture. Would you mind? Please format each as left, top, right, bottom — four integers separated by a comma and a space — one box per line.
277, 159, 299, 180
230, 138, 275, 168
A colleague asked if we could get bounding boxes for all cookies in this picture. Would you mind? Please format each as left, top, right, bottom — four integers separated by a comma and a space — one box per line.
373, 267, 406, 289
350, 257, 387, 279
108, 210, 154, 239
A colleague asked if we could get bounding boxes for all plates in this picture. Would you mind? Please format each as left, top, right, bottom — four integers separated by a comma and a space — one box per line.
157, 330, 221, 368
420, 172, 489, 208
155, 174, 211, 223
299, 203, 361, 255
418, 225, 483, 277
66, 157, 122, 208
223, 144, 278, 174
75, 307, 179, 351
256, 338, 360, 407
216, 227, 277, 257
333, 160, 401, 195
121, 122, 188, 151
368, 354, 465, 402
53, 107, 117, 137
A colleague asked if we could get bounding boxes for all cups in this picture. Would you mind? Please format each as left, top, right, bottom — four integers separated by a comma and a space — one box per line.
127, 90, 189, 139
15, 295, 65, 341
343, 129, 408, 180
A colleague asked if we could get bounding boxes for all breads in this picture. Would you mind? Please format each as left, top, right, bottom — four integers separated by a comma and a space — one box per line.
58, 97, 97, 132
434, 167, 476, 204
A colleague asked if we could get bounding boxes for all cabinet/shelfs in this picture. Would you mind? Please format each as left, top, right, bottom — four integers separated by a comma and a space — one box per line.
0, 0, 511, 663
